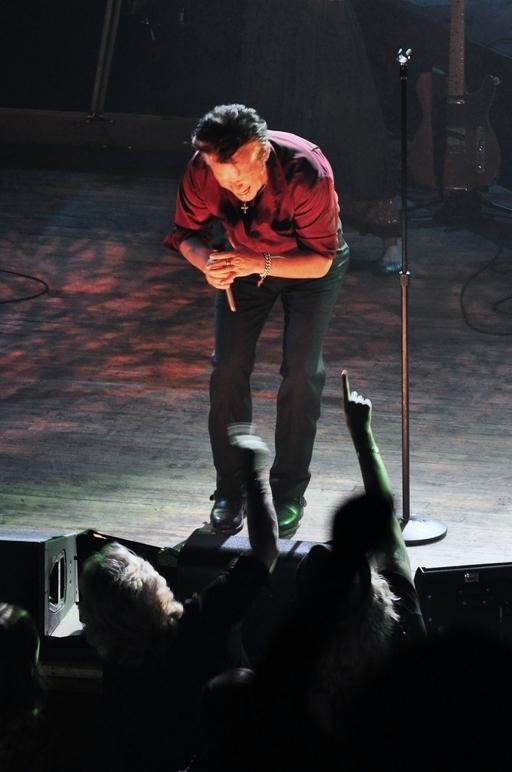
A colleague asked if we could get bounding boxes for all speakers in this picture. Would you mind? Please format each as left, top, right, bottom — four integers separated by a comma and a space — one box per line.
414, 560, 512, 641
174, 531, 334, 608
0, 530, 76, 637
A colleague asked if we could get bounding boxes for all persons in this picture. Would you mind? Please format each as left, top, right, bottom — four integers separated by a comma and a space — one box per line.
162, 103, 351, 538
1, 369, 511, 771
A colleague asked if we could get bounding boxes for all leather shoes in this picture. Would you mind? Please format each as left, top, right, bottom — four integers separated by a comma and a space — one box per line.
209, 488, 245, 537
273, 496, 304, 538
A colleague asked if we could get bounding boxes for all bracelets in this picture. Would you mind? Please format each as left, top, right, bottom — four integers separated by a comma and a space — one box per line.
261, 252, 272, 276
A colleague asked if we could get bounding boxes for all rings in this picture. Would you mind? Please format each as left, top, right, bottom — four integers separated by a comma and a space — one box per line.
226, 260, 231, 266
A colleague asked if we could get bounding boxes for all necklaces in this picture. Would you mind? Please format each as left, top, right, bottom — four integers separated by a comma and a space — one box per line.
240, 202, 249, 214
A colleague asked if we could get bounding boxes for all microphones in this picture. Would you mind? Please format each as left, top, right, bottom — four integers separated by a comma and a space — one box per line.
208, 237, 237, 314
